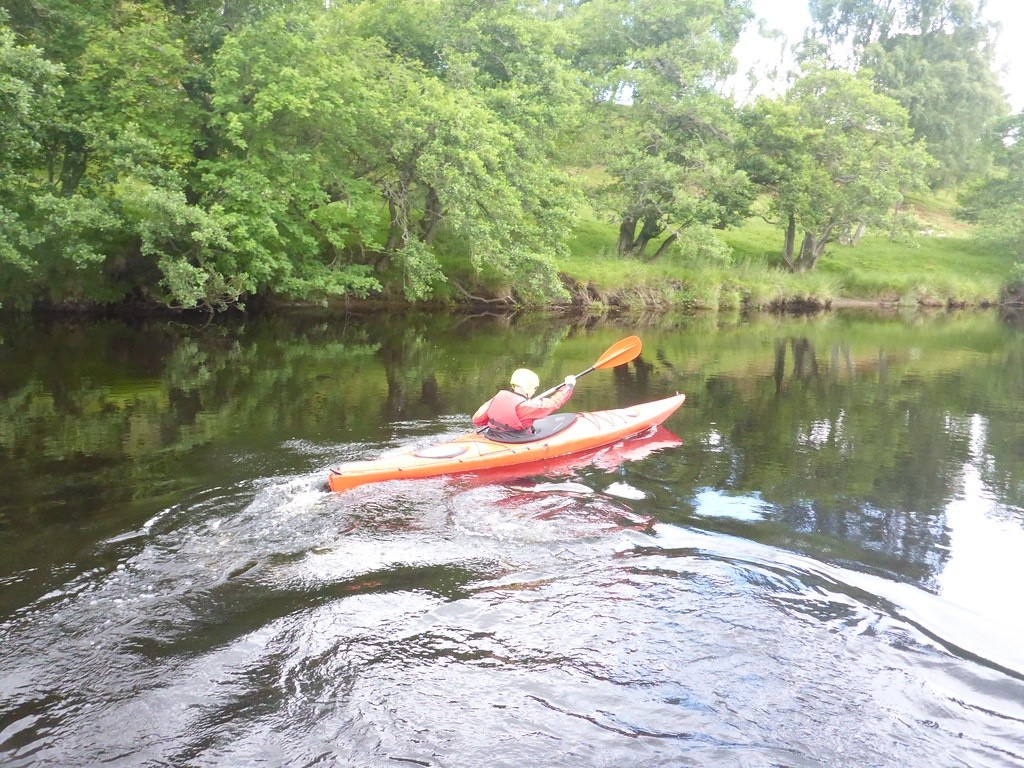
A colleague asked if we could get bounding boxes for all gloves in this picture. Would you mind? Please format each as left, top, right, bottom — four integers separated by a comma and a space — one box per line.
565, 375, 576, 390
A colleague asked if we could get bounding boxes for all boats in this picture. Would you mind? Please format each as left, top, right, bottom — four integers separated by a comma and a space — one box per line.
327, 391, 685, 493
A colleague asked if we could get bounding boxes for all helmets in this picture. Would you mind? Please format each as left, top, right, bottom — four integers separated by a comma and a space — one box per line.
510, 369, 539, 399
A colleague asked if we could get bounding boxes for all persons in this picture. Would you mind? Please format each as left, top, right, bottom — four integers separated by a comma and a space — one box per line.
472, 368, 578, 433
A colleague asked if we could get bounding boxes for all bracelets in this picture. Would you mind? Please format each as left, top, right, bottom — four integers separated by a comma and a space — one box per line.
566, 384, 574, 388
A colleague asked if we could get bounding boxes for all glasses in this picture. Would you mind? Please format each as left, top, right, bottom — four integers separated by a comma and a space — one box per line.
534, 387, 537, 391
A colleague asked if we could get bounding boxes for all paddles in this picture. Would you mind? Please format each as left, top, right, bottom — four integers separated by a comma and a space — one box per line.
474, 336, 641, 434
